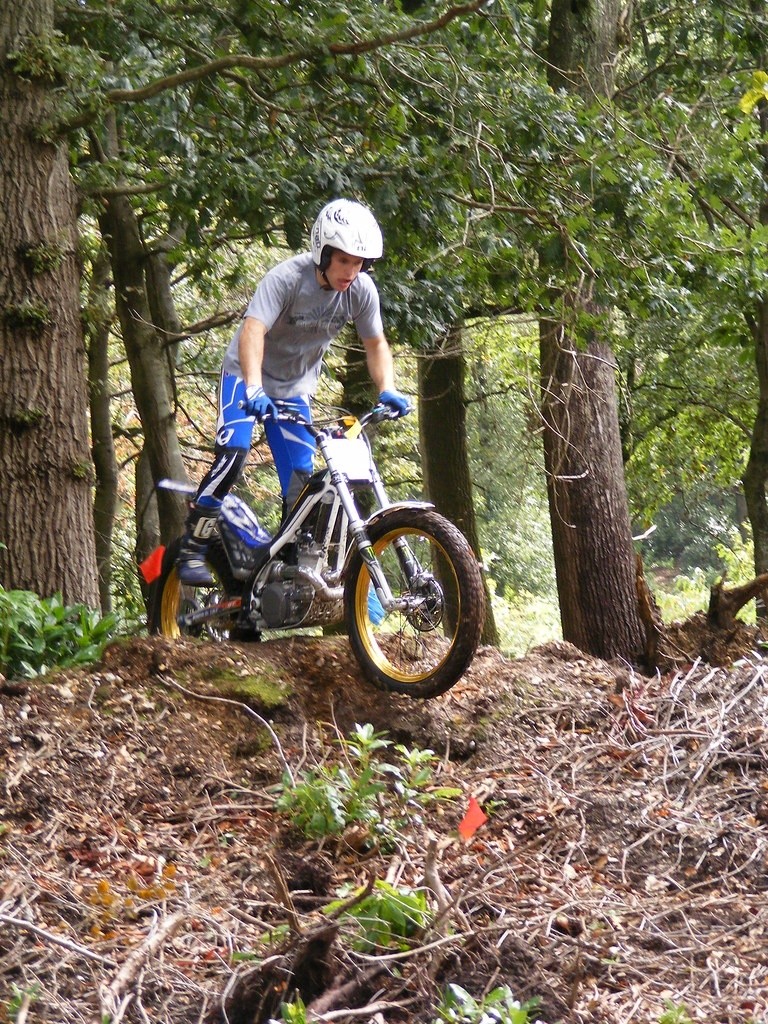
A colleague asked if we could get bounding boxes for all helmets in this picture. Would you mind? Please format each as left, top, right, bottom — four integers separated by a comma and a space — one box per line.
310, 199, 384, 272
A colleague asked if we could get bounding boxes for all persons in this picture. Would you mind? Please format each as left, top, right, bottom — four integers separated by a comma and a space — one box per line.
177, 198, 409, 586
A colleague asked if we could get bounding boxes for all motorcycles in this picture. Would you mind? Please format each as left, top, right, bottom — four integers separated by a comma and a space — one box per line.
139, 392, 485, 701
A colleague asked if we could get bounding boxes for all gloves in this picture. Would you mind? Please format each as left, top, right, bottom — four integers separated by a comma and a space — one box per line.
379, 389, 410, 421
244, 386, 277, 424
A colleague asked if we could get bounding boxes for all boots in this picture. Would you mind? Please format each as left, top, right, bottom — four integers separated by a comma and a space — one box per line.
177, 501, 221, 586
279, 501, 297, 564
